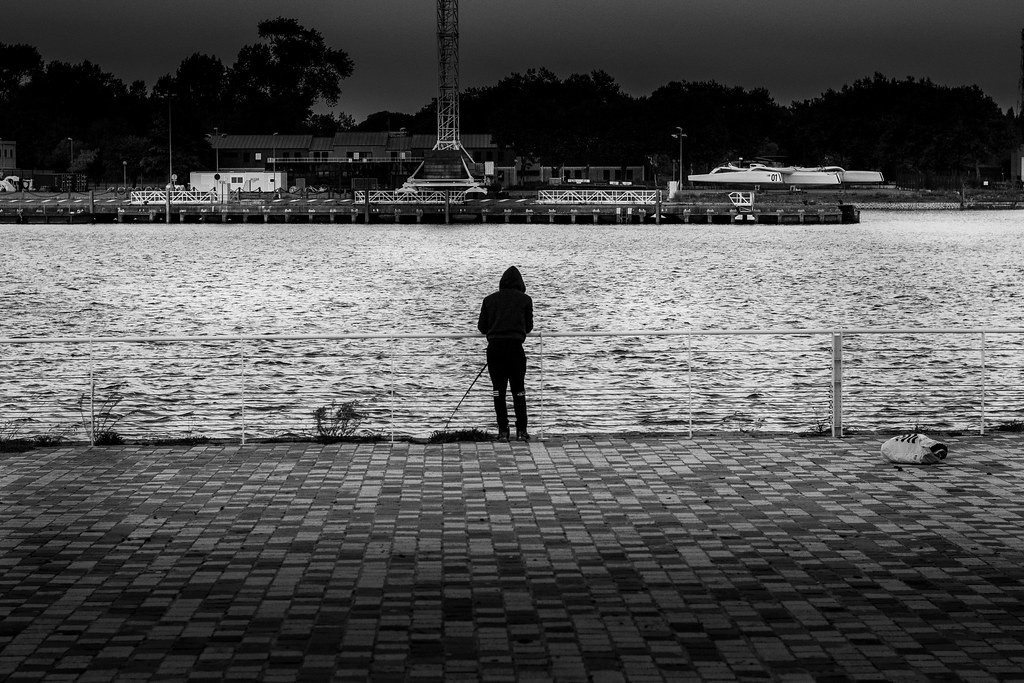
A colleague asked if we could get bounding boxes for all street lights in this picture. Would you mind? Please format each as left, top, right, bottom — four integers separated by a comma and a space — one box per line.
68, 137, 74, 174
164, 91, 178, 183
122, 161, 128, 188
272, 133, 279, 192
671, 126, 688, 192
206, 128, 228, 175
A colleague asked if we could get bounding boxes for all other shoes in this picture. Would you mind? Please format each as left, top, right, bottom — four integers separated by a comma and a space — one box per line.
517, 432, 530, 440
497, 433, 510, 441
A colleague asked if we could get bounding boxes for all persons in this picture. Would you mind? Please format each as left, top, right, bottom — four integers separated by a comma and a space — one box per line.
477, 266, 535, 442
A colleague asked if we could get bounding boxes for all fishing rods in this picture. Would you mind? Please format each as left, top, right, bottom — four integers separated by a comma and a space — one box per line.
441, 365, 491, 433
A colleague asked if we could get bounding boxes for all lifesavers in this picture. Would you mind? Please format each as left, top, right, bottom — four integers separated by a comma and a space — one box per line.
117, 186, 126, 195
106, 187, 114, 193
128, 186, 162, 192
